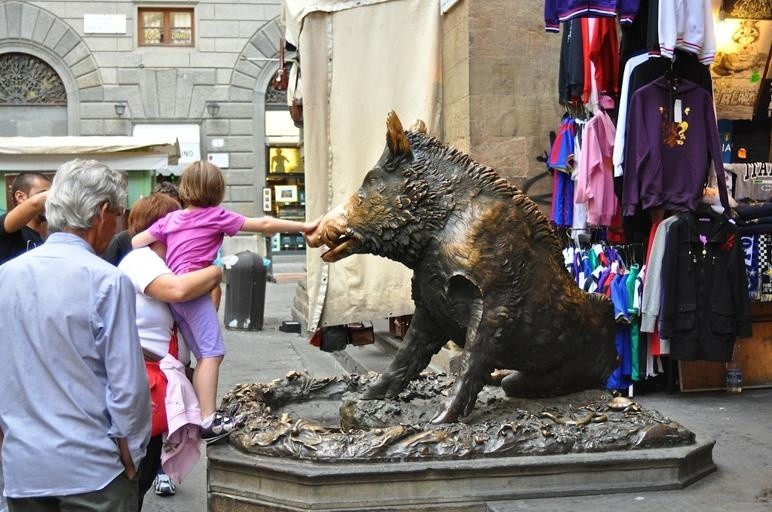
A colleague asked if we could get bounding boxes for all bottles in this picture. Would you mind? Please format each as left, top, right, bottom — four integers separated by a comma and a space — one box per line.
725, 345, 743, 392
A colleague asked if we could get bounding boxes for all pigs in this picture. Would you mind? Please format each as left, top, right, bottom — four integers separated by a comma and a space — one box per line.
304, 110, 618, 425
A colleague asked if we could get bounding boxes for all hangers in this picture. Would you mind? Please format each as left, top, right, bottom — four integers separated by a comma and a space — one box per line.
695, 187, 721, 220
561, 90, 607, 128
560, 225, 647, 271
663, 54, 681, 82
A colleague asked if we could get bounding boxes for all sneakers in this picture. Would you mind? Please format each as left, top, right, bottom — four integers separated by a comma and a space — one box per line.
153, 473, 176, 494
199, 415, 247, 444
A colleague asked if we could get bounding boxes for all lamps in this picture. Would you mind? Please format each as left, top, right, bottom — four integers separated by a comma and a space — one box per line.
206, 103, 220, 117
114, 102, 125, 117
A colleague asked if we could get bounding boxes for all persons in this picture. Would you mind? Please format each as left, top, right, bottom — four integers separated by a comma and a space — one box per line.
0, 170, 53, 266
117, 193, 221, 511
102, 181, 183, 495
130, 160, 323, 445
0, 159, 148, 512
